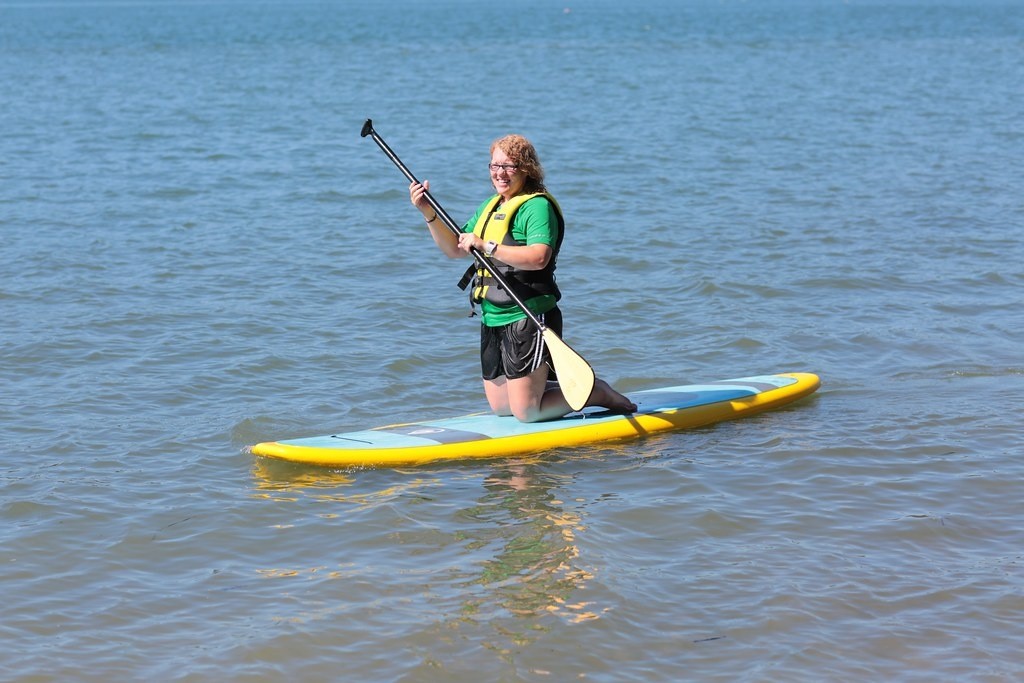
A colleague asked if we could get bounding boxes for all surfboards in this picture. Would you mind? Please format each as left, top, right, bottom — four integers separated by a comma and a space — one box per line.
250, 371, 821, 470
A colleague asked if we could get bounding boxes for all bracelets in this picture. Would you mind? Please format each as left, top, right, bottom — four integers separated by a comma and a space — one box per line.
425, 212, 437, 223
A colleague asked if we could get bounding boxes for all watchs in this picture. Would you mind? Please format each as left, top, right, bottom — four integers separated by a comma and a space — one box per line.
485, 239, 498, 257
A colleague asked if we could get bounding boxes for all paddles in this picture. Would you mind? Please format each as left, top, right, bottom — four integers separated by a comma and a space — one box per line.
359, 118, 596, 412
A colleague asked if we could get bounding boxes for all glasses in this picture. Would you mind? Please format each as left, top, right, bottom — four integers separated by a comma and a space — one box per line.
488, 163, 523, 172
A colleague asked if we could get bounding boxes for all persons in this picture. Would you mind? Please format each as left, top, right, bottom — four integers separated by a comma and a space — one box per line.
408, 134, 638, 423
